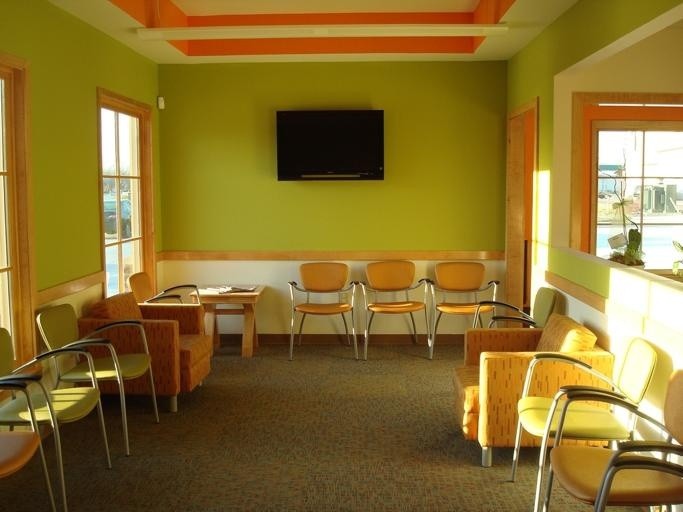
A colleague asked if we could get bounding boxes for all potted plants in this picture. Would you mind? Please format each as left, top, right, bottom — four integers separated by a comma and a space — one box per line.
598, 171, 644, 271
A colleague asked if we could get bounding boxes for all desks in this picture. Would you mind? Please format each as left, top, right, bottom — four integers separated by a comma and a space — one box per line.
189, 285, 266, 358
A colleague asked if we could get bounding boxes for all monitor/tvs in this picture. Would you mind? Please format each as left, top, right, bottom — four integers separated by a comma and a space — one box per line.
275, 109, 385, 182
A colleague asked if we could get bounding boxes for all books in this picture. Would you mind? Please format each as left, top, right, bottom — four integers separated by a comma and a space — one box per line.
204, 285, 256, 294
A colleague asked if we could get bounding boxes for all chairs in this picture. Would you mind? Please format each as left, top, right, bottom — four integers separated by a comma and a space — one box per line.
126, 272, 201, 304
473, 287, 558, 328
287, 261, 500, 359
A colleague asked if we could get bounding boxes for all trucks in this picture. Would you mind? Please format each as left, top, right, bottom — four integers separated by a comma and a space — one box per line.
101, 170, 131, 239
